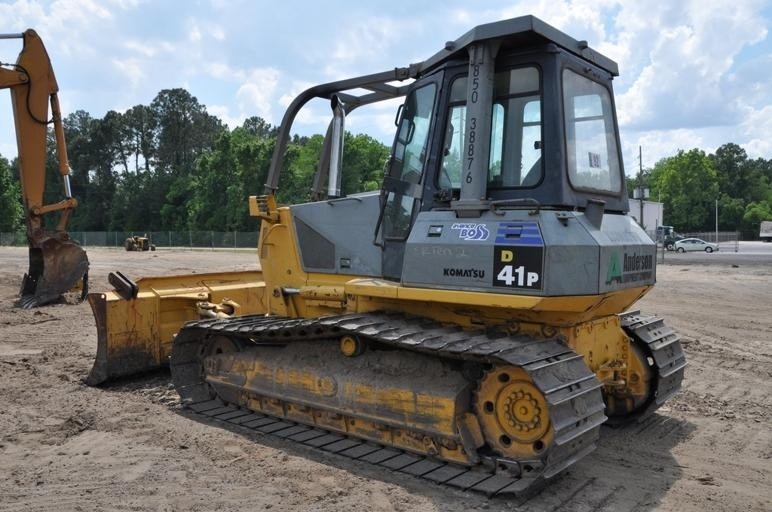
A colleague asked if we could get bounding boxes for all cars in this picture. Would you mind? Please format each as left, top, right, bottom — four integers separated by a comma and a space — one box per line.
664, 236, 719, 253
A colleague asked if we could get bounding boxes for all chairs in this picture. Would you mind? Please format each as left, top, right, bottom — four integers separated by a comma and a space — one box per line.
520, 156, 541, 186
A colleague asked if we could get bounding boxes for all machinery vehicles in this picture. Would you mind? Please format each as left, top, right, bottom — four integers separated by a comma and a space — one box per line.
126, 234, 155, 251
84, 11, 690, 500
0, 29, 89, 305
